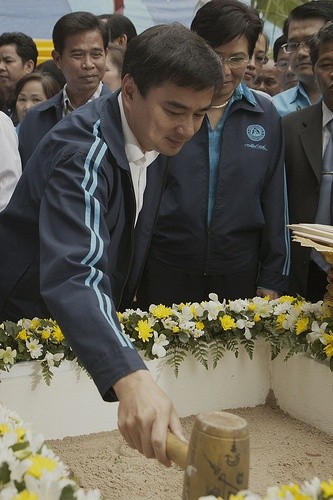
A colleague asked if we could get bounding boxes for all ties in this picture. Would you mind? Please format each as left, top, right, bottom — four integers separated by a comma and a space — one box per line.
311, 119, 333, 274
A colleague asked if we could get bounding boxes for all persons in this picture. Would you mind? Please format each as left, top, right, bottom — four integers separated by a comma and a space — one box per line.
1, 1, 333, 467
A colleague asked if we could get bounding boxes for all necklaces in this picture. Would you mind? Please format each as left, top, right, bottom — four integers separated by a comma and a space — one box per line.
210, 98, 230, 109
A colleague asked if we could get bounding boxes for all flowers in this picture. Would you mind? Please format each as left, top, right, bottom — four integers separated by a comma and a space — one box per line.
0, 290, 333, 500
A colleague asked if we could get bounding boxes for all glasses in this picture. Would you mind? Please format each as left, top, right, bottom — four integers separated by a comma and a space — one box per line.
281, 41, 310, 53
220, 55, 249, 68
255, 55, 269, 65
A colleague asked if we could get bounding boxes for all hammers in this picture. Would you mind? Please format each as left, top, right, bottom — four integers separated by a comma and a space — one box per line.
166, 410, 250, 500
